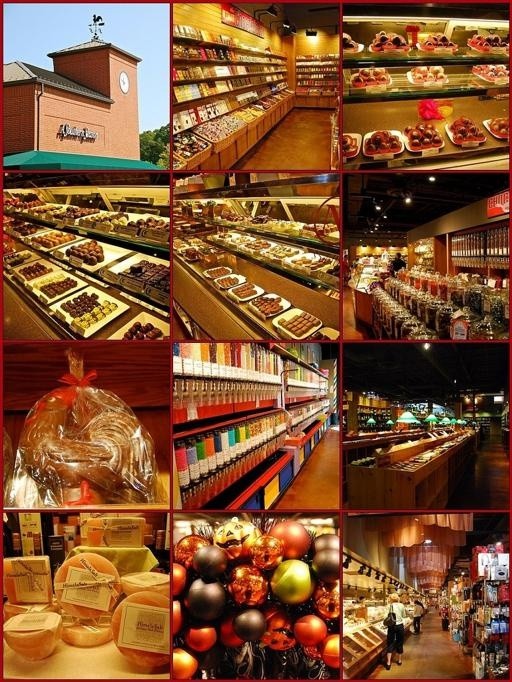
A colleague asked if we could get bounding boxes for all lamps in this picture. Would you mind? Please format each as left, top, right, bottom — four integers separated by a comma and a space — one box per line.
269, 17, 293, 31
396, 408, 418, 424
386, 415, 393, 425
251, 4, 280, 21
305, 27, 318, 37
366, 411, 376, 424
442, 416, 450, 424
423, 411, 438, 423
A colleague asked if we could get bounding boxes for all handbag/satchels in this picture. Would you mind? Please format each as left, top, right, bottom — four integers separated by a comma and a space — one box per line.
384, 604, 396, 628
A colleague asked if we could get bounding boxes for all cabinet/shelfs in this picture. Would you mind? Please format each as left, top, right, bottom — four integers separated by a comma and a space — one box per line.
349, 426, 477, 507
171, 341, 332, 506
171, 23, 294, 172
343, 614, 415, 677
439, 568, 509, 680
349, 259, 380, 327
340, 13, 510, 170
296, 55, 339, 109
2, 185, 171, 342
172, 197, 340, 343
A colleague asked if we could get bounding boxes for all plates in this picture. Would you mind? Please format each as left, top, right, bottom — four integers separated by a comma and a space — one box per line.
406, 71, 449, 85
248, 293, 291, 317
285, 252, 336, 273
343, 133, 363, 159
415, 43, 459, 53
343, 43, 365, 54
467, 43, 507, 55
445, 123, 487, 145
227, 282, 265, 302
363, 130, 404, 157
202, 266, 233, 279
272, 308, 322, 340
482, 118, 510, 139
368, 43, 412, 54
349, 73, 392, 88
213, 273, 247, 289
473, 71, 509, 83
402, 129, 446, 152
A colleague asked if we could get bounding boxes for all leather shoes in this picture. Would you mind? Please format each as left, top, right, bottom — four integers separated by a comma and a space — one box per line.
413, 631, 419, 635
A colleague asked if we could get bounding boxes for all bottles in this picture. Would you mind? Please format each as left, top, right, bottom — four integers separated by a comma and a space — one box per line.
195, 437, 209, 475
205, 433, 217, 472
175, 440, 190, 489
237, 411, 289, 455
172, 342, 285, 376
186, 440, 200, 480
221, 430, 230, 462
215, 431, 226, 469
228, 427, 236, 459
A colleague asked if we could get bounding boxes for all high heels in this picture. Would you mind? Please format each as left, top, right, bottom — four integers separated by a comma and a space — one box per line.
397, 660, 402, 666
384, 662, 391, 671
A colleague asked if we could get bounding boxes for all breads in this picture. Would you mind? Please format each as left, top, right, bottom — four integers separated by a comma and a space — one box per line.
60, 620, 111, 647
66, 240, 105, 266
112, 592, 170, 670
6, 387, 166, 508
4, 555, 52, 603
79, 211, 169, 241
62, 204, 99, 220
87, 518, 146, 548
55, 553, 119, 619
121, 572, 171, 598
31, 232, 76, 247
4, 613, 62, 659
4, 595, 57, 621
117, 259, 170, 293
60, 291, 118, 332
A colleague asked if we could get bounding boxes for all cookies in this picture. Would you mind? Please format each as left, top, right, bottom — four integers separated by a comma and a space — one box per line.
231, 283, 258, 298
277, 310, 322, 338
251, 296, 286, 314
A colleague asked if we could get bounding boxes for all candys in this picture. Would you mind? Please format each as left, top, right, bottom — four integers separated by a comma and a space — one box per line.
174, 519, 340, 681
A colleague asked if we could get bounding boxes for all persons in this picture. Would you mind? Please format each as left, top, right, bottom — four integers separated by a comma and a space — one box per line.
385, 593, 408, 670
412, 599, 425, 636
